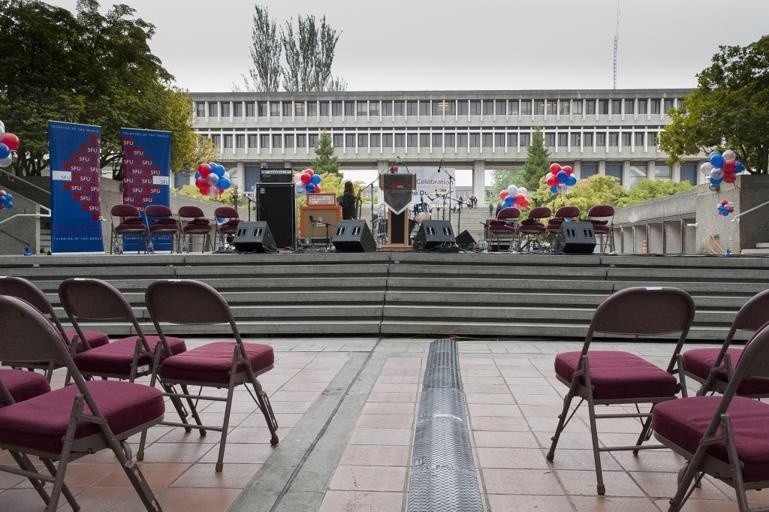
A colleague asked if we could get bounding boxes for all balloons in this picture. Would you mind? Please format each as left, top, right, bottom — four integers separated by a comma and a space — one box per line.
728, 208, 735, 213
207, 185, 220, 198
0, 120, 5, 128
194, 170, 202, 179
0, 196, 7, 202
0, 127, 5, 141
300, 172, 311, 186
6, 201, 15, 208
296, 190, 307, 195
723, 210, 728, 215
293, 172, 302, 183
557, 182, 566, 192
720, 199, 728, 205
199, 163, 211, 179
196, 177, 210, 189
507, 185, 517, 195
198, 187, 212, 196
546, 175, 560, 186
513, 194, 525, 203
0, 189, 6, 196
305, 182, 315, 192
699, 160, 712, 175
501, 203, 512, 208
716, 203, 721, 208
563, 166, 572, 175
708, 150, 722, 159
204, 172, 219, 187
709, 154, 723, 166
215, 164, 225, 177
519, 200, 530, 208
721, 159, 734, 171
558, 169, 568, 182
727, 201, 735, 207
312, 184, 321, 193
295, 182, 304, 192
504, 196, 515, 203
3, 132, 19, 150
720, 148, 735, 160
217, 188, 225, 194
209, 161, 217, 170
518, 187, 528, 195
546, 171, 553, 180
563, 185, 574, 194
719, 211, 723, 215
733, 159, 745, 174
708, 177, 725, 186
0, 203, 5, 209
723, 171, 736, 183
6, 193, 13, 201
498, 189, 510, 198
217, 177, 231, 189
564, 176, 576, 186
549, 185, 558, 195
549, 163, 562, 175
0, 143, 10, 159
1, 153, 12, 167
305, 168, 315, 176
724, 203, 729, 210
720, 206, 724, 210
708, 168, 726, 180
309, 174, 322, 185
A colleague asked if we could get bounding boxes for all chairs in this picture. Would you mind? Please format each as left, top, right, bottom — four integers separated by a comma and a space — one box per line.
143, 205, 180, 252
136, 279, 279, 473
1, 362, 79, 512
180, 207, 213, 253
0, 291, 167, 512
1, 277, 111, 385
214, 208, 243, 253
587, 207, 615, 253
651, 322, 768, 512
108, 204, 147, 253
490, 208, 521, 251
648, 290, 769, 487
517, 207, 550, 252
548, 285, 695, 496
545, 208, 580, 254
58, 275, 208, 460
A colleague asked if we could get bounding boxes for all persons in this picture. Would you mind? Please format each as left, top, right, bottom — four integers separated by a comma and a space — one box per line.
473, 196, 477, 205
496, 201, 502, 211
459, 195, 464, 209
470, 195, 474, 206
489, 202, 493, 217
340, 181, 357, 220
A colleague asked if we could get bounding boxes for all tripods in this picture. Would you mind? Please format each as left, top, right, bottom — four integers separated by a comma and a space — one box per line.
315, 218, 337, 249
426, 189, 442, 219
447, 194, 477, 234
432, 190, 454, 219
442, 168, 456, 223
361, 168, 388, 234
241, 194, 257, 221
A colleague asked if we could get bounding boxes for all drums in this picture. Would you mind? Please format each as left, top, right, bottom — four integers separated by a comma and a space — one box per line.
373, 218, 388, 239
414, 202, 431, 223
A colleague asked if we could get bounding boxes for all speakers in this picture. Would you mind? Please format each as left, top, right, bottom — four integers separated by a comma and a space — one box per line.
257, 183, 295, 249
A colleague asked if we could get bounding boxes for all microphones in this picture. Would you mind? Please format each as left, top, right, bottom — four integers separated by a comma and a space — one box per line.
438, 162, 440, 172
309, 215, 314, 225
396, 155, 410, 174
